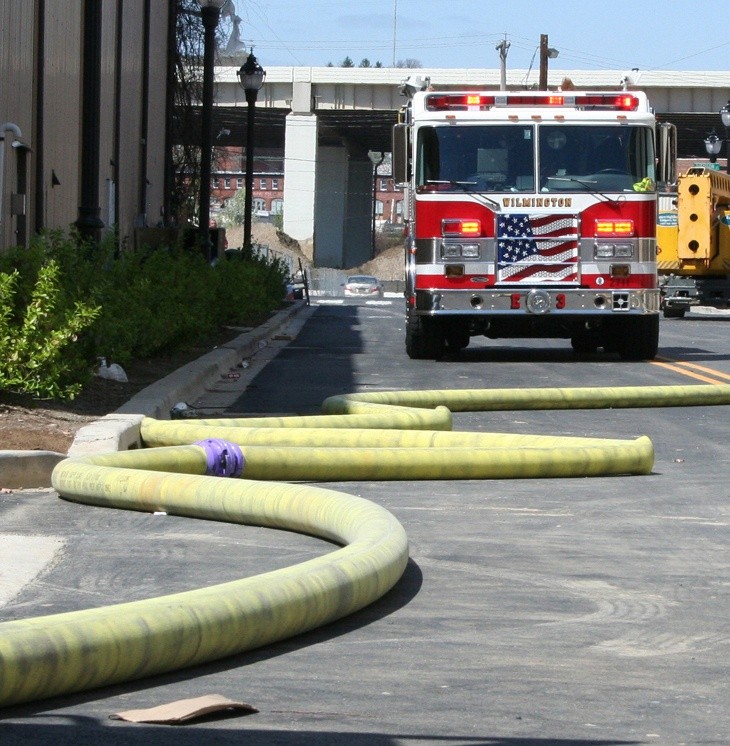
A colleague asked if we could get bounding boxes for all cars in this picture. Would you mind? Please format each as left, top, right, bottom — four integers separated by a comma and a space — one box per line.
340, 275, 385, 301
382, 213, 407, 238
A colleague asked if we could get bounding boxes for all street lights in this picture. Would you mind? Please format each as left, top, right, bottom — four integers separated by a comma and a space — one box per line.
237, 46, 266, 251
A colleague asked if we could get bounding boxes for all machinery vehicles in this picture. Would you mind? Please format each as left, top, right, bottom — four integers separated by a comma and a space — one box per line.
657, 162, 730, 317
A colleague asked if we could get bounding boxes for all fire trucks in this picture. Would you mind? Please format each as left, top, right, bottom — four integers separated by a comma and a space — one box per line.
391, 33, 678, 361
